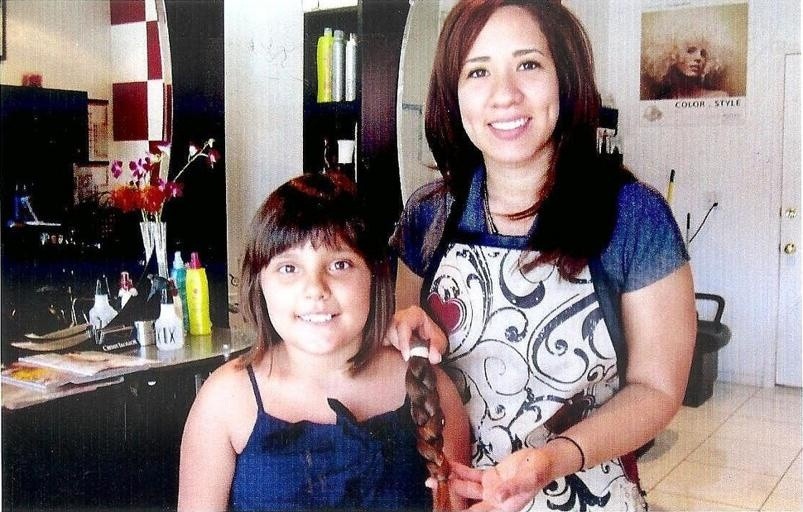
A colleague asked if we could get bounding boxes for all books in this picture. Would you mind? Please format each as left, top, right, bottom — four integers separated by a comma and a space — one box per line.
1, 350, 164, 411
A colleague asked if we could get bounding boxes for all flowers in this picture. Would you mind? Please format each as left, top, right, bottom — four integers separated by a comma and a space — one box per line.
109, 140, 172, 222
135, 138, 220, 223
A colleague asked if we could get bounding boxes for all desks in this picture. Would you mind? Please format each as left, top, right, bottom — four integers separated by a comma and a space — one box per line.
1, 328, 257, 512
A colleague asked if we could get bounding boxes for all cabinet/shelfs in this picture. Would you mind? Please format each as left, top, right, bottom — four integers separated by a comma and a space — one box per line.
304, 3, 409, 222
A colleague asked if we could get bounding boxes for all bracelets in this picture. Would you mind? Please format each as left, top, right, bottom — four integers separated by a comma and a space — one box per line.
545, 434, 587, 471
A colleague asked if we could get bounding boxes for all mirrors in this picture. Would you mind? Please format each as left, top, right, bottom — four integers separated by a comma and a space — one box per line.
0, 1, 174, 352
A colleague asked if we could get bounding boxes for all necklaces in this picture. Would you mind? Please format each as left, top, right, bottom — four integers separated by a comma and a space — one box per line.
482, 181, 500, 234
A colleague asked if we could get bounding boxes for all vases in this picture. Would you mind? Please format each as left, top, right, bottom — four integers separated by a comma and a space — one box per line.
140, 222, 154, 266
153, 223, 170, 280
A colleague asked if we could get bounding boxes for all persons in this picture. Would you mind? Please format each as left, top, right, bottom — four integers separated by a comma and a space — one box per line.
382, 1, 697, 510
644, 25, 729, 99
177, 169, 472, 511
